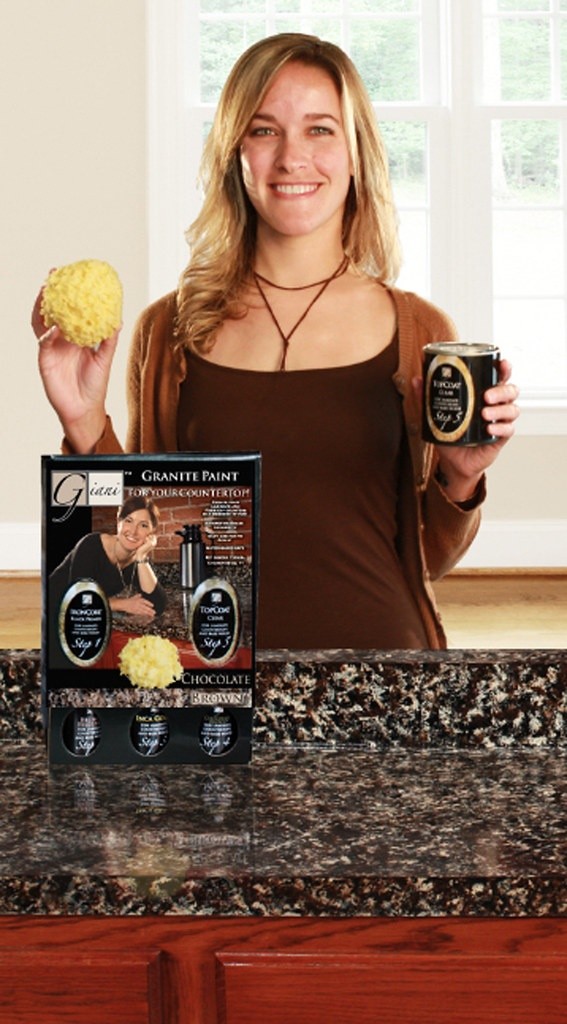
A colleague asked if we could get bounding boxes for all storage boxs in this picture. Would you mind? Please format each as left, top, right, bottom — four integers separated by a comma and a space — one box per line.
40, 452, 261, 766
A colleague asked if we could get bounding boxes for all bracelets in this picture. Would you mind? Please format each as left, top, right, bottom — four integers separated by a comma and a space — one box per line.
136, 558, 149, 563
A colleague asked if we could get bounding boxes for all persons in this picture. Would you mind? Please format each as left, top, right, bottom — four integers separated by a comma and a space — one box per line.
30, 33, 518, 650
49, 498, 167, 635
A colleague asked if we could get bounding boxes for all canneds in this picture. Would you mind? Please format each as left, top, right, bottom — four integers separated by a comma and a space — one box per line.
420, 341, 502, 448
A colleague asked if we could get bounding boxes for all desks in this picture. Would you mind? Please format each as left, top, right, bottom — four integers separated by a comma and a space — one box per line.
0, 740, 567, 1024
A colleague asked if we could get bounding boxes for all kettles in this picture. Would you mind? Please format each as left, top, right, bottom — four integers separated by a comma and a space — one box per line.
174, 523, 214, 590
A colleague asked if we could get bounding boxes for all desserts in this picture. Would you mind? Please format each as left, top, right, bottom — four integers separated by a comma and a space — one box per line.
118, 633, 183, 690
39, 259, 123, 347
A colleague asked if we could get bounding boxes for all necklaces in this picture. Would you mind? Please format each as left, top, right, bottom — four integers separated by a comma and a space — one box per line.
114, 539, 137, 601
